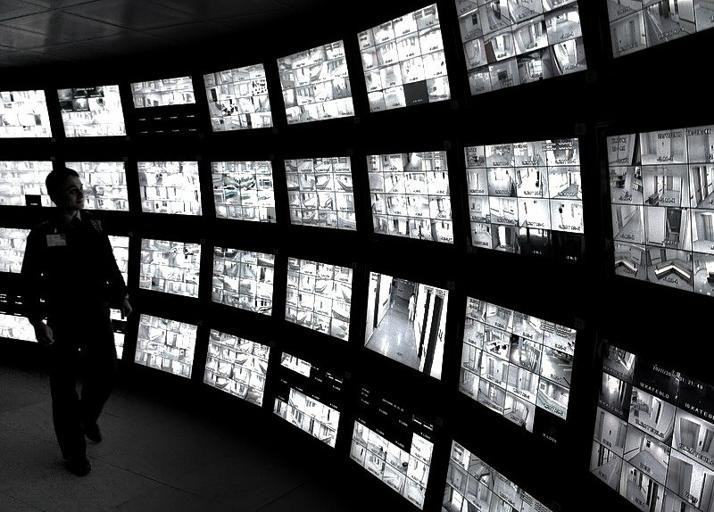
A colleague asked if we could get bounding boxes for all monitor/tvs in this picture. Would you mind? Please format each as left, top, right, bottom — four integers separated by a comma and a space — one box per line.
1, 161, 57, 208
607, 0, 714, 58
364, 271, 450, 382
211, 160, 277, 223
356, 3, 451, 111
203, 63, 273, 128
0, 294, 47, 343
106, 235, 130, 286
110, 308, 127, 360
454, 0, 588, 95
285, 256, 354, 341
277, 39, 355, 124
463, 137, 585, 263
137, 161, 203, 216
458, 297, 577, 446
285, 156, 357, 231
0, 228, 31, 273
57, 85, 127, 138
350, 386, 439, 511
272, 352, 345, 449
134, 314, 198, 379
64, 161, 129, 212
0, 89, 53, 138
588, 346, 714, 512
129, 75, 200, 134
203, 328, 272, 408
608, 125, 714, 297
441, 439, 553, 512
367, 151, 454, 244
139, 238, 202, 299
212, 246, 275, 317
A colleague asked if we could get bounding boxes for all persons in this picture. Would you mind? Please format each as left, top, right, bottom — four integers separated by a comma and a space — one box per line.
19, 167, 137, 478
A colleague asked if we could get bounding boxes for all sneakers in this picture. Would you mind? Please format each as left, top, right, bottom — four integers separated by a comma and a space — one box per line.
65, 452, 91, 476
84, 420, 102, 443
